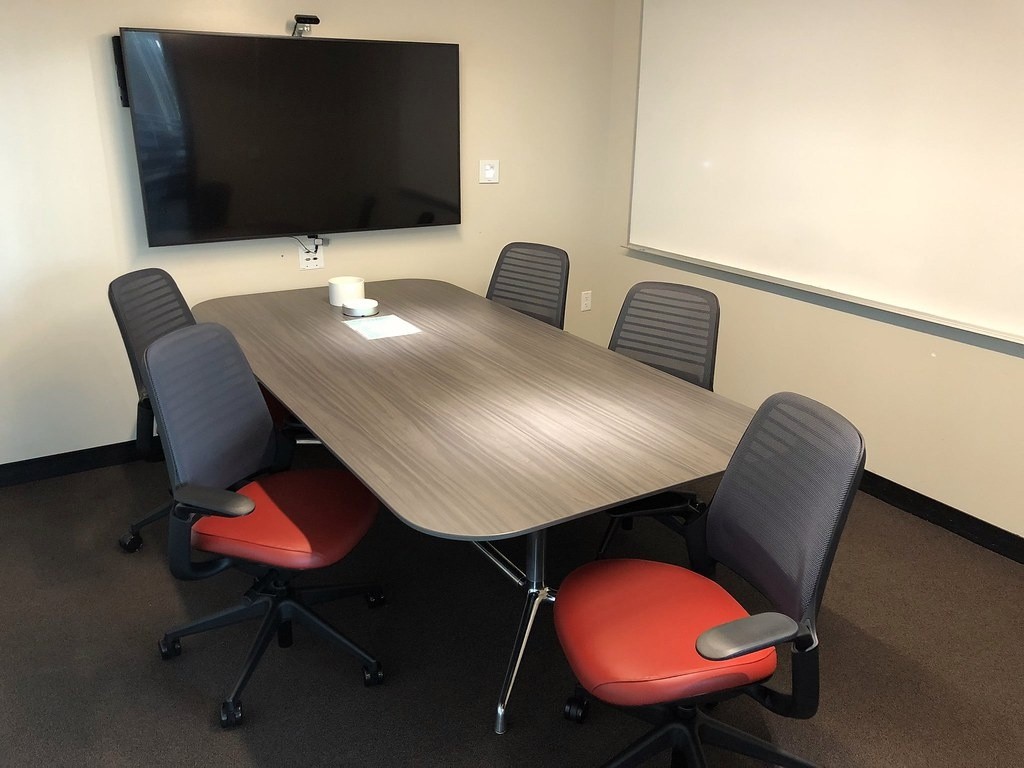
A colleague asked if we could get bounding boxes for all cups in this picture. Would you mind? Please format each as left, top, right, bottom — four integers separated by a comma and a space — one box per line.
329, 276, 365, 307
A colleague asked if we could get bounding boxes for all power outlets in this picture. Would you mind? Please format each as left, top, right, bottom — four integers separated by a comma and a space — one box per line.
581, 291, 592, 312
299, 246, 323, 270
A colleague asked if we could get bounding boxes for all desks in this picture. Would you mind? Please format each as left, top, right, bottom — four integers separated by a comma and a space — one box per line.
192, 278, 756, 736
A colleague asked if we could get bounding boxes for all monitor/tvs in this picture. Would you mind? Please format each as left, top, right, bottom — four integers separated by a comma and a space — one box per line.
119, 27, 462, 248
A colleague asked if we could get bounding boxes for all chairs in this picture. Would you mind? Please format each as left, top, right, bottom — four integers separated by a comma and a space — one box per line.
486, 241, 571, 330
142, 322, 392, 728
554, 390, 868, 768
108, 268, 296, 553
579, 280, 720, 564
